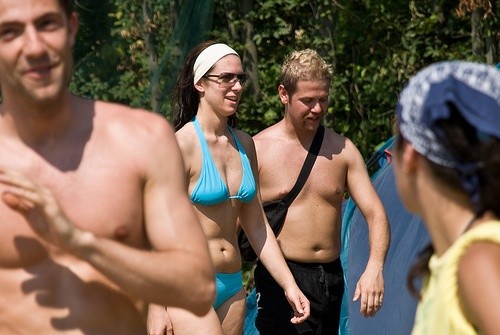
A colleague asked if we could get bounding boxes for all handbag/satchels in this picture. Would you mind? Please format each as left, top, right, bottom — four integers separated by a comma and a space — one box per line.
238, 198, 290, 263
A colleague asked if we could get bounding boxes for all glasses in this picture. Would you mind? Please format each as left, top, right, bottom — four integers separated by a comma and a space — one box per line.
201, 72, 247, 87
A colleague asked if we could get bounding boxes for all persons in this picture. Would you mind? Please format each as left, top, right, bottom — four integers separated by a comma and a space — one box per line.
237, 49, 390, 335
145, 40, 311, 335
392, 61, 500, 335
0, 0, 215, 335
